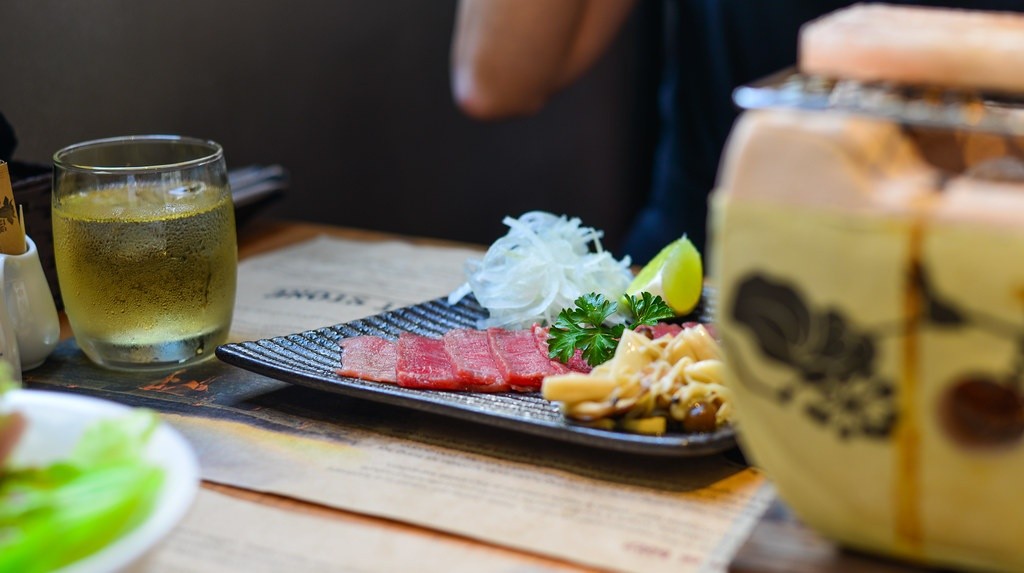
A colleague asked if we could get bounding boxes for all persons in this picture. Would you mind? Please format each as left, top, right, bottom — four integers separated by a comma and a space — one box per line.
448, 0, 1024, 269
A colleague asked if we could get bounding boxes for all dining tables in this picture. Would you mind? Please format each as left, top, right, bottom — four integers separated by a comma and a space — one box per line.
2, 137, 929, 571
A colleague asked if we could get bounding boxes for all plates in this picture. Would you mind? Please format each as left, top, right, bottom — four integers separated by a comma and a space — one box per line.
1, 389, 199, 573
213, 286, 736, 456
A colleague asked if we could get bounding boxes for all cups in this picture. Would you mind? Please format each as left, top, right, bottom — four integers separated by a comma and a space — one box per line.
51, 135, 239, 374
0, 232, 61, 377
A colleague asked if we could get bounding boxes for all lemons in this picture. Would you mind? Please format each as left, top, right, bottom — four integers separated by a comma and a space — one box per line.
621, 231, 704, 318
